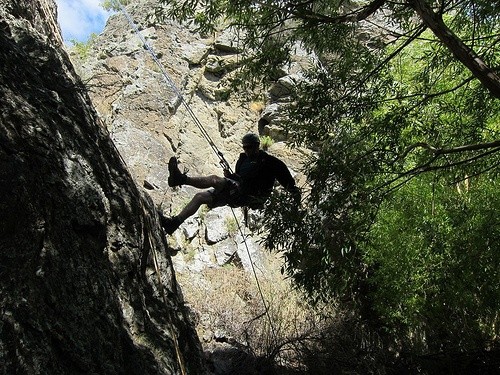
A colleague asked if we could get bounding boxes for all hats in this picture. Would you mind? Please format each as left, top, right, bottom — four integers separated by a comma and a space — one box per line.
242, 134, 260, 144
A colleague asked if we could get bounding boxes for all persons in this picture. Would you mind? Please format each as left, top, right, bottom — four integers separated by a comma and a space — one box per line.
158, 134, 302, 235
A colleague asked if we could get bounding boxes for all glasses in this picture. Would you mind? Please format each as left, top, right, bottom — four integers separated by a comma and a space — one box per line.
242, 145, 258, 150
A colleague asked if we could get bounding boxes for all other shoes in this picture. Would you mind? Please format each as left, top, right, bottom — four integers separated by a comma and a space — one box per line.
156, 211, 181, 235
168, 157, 188, 188
